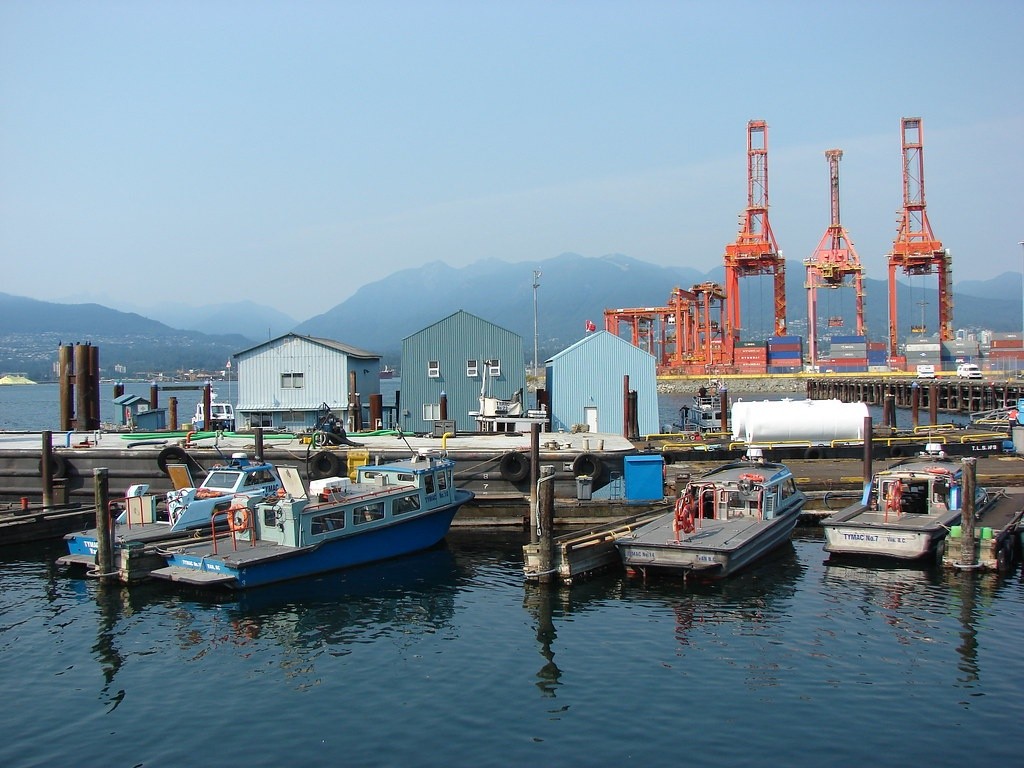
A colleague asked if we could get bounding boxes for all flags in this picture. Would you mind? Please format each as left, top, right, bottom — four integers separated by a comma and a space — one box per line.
586, 320, 596, 334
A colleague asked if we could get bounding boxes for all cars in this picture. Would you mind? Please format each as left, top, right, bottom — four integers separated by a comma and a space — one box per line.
918, 369, 935, 380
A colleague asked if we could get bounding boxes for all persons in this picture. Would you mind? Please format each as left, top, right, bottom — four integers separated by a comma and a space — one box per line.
699, 385, 708, 401
679, 404, 692, 424
712, 380, 721, 392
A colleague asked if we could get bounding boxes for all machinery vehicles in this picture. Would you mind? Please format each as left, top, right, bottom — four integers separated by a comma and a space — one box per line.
301, 402, 348, 447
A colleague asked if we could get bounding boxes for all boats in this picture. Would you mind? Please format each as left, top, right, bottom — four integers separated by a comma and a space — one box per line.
684, 377, 732, 434
819, 443, 988, 560
614, 447, 807, 585
57, 433, 476, 590
190, 376, 235, 431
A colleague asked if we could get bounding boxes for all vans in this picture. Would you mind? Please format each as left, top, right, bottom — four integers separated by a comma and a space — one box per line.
956, 363, 982, 380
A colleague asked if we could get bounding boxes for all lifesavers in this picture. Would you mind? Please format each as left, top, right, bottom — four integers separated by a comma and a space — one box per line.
892, 486, 900, 512
311, 451, 339, 478
682, 504, 696, 534
157, 446, 187, 473
197, 489, 221, 497
571, 453, 601, 478
499, 452, 529, 481
227, 502, 249, 532
922, 465, 950, 475
37, 453, 64, 478
738, 473, 765, 482
